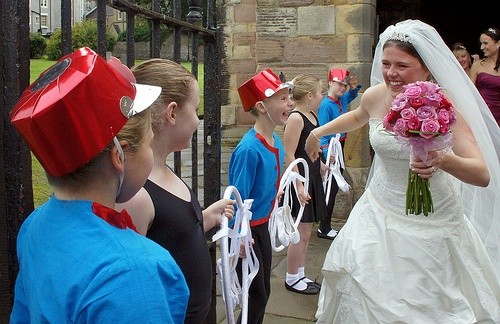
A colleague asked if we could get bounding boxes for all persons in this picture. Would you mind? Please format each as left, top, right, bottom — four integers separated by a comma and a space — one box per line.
113, 59, 237, 324
227, 68, 294, 324
450, 27, 500, 128
9, 47, 190, 324
278, 73, 322, 295
306, 19, 500, 324
316, 69, 364, 240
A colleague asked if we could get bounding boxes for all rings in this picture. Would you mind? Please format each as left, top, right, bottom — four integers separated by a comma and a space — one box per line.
432, 167, 436, 172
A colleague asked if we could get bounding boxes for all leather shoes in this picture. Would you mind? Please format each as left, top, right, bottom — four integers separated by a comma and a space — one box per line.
285, 277, 320, 296
317, 228, 337, 237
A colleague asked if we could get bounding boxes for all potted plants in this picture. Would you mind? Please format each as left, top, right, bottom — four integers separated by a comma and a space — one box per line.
106, 35, 115, 60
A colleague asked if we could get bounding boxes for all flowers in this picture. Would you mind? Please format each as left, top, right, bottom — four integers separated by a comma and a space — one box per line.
381, 80, 457, 216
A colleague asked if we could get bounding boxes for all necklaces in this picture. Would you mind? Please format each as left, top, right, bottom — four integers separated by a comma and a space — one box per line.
383, 95, 389, 113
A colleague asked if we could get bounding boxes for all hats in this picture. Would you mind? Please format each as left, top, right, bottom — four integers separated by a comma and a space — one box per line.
14, 47, 162, 176
238, 70, 292, 113
328, 69, 350, 86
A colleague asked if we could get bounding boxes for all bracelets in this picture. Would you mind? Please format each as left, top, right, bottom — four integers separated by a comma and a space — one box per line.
310, 130, 321, 142
